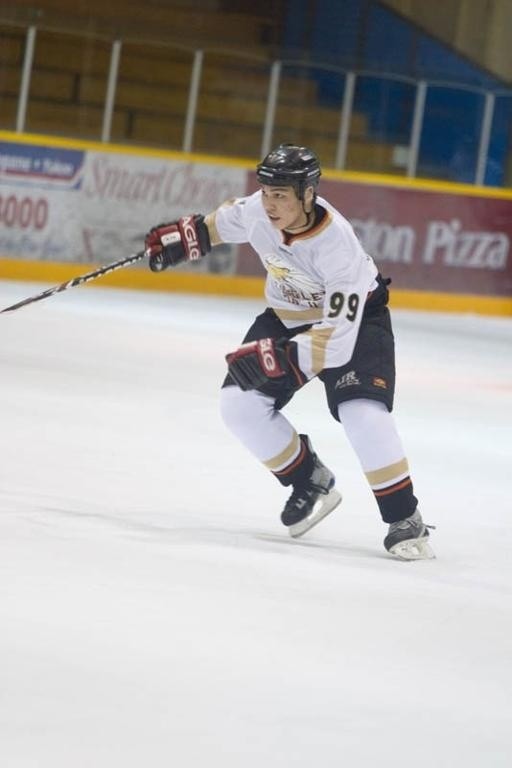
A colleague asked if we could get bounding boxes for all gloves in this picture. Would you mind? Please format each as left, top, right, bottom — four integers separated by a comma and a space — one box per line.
224, 335, 290, 392
142, 211, 211, 273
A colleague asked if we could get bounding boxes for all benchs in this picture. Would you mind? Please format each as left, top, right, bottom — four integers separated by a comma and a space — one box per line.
0, 1, 400, 175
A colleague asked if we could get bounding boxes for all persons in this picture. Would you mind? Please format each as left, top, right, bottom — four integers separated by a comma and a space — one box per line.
145, 143, 433, 551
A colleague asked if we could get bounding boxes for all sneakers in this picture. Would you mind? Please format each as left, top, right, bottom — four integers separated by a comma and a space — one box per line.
280, 451, 335, 526
383, 506, 430, 552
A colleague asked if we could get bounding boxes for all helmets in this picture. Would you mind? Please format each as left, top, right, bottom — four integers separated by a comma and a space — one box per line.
254, 140, 323, 204
